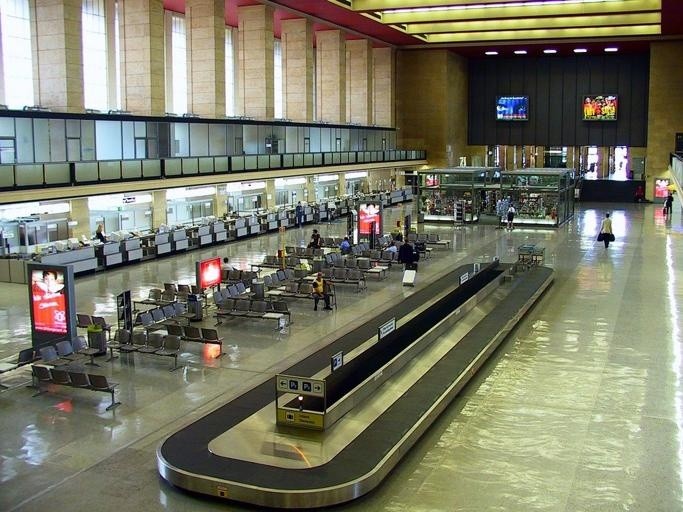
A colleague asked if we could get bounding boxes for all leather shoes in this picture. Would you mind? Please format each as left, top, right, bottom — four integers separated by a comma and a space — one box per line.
314, 307, 317, 311
324, 306, 332, 310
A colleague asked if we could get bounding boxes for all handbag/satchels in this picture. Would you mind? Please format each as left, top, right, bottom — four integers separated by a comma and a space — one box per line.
405, 263, 418, 272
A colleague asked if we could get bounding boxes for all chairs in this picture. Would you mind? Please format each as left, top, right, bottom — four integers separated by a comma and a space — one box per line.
3, 307, 228, 412
130, 234, 450, 332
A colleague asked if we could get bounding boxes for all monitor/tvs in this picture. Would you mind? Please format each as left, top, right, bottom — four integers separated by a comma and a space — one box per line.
494, 94, 530, 122
582, 94, 619, 122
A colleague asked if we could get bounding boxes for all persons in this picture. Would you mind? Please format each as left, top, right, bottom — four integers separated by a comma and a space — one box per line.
600, 212, 612, 248
397, 239, 412, 272
220, 257, 236, 270
95, 224, 106, 243
338, 236, 350, 254
294, 200, 302, 228
665, 193, 673, 213
312, 271, 334, 311
634, 185, 643, 202
31, 271, 66, 294
306, 230, 322, 248
506, 203, 515, 230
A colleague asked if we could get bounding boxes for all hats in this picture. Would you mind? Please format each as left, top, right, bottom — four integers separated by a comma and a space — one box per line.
318, 272, 322, 278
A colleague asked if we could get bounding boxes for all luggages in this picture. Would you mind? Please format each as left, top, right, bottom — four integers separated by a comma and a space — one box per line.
401, 262, 417, 287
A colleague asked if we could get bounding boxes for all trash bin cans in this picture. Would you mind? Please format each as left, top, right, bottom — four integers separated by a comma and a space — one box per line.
188, 299, 203, 322
313, 259, 324, 273
250, 282, 265, 299
362, 250, 372, 257
88, 325, 107, 356
